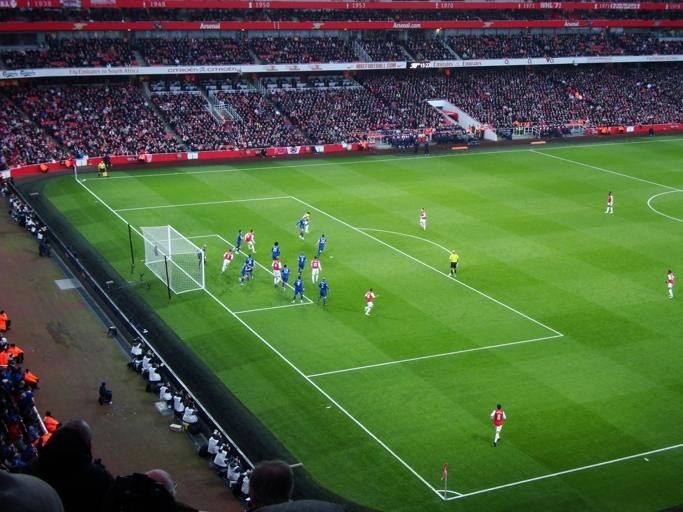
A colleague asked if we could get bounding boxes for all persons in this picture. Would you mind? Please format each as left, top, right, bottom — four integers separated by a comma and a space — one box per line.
0, 305, 294, 511
488, 404, 506, 446
604, 190, 614, 214
447, 249, 458, 278
1, 176, 50, 258
418, 208, 427, 231
364, 287, 379, 317
0, 0, 682, 179
663, 268, 676, 299
196, 210, 331, 307
